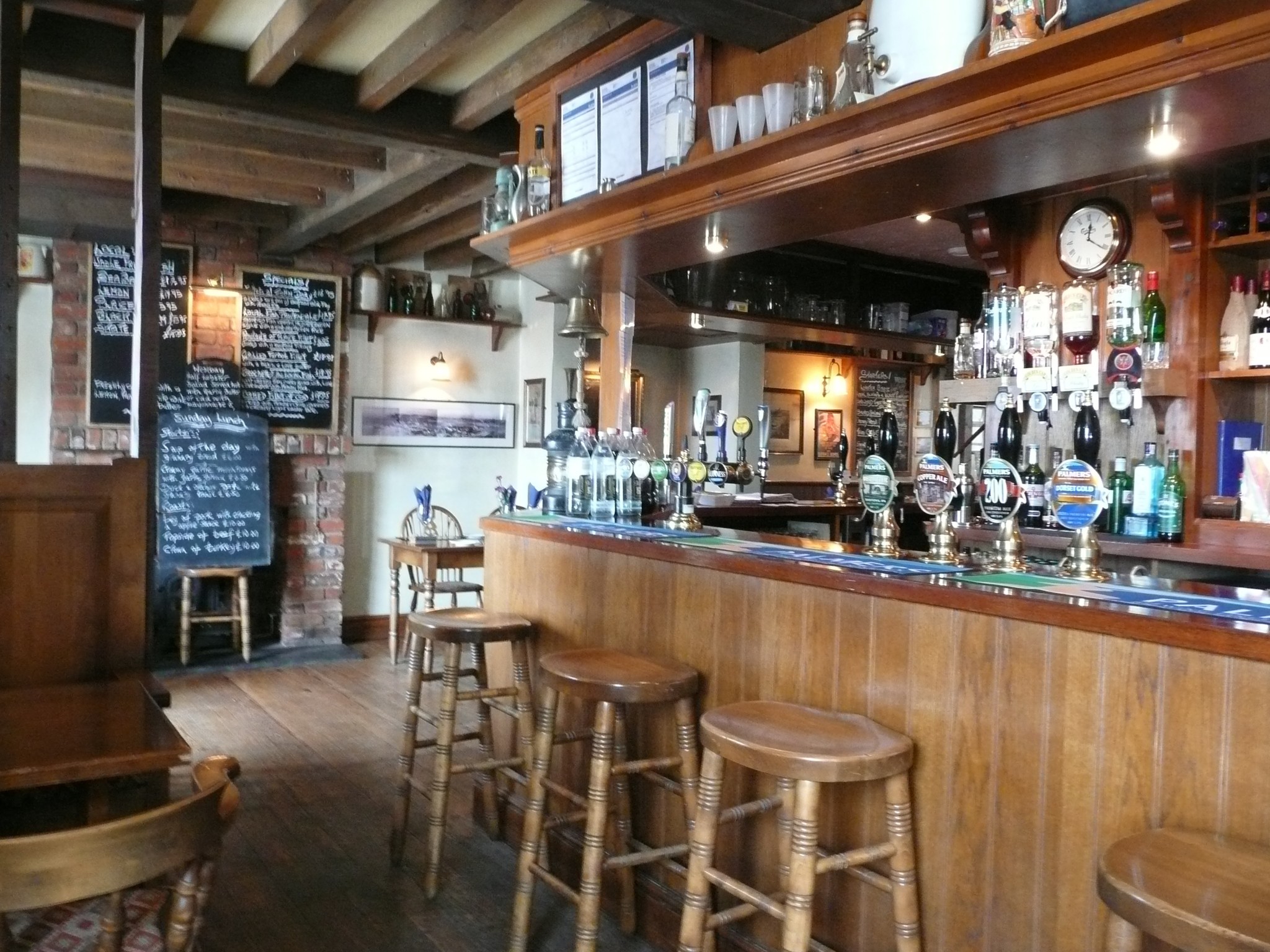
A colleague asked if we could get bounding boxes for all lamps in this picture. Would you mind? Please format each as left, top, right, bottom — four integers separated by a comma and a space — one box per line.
822, 358, 848, 398
431, 351, 452, 382
1144, 122, 1189, 157
687, 313, 706, 329
703, 235, 728, 253
188, 267, 255, 299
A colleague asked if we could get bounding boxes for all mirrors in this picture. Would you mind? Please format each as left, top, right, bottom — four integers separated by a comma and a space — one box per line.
582, 376, 637, 439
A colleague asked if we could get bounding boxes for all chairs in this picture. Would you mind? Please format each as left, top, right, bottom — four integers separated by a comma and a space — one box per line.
0, 754, 244, 952
400, 506, 484, 660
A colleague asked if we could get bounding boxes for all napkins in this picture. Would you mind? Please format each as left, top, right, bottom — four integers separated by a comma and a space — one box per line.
528, 482, 549, 511
414, 483, 432, 521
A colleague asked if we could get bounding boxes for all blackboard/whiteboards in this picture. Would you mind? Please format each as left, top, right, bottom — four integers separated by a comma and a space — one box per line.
88, 240, 195, 427
155, 411, 274, 566
233, 263, 343, 435
185, 359, 243, 414
850, 362, 915, 478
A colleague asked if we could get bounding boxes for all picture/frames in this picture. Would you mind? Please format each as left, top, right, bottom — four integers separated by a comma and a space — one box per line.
763, 387, 805, 456
914, 436, 934, 456
914, 409, 934, 430
692, 394, 722, 437
813, 408, 843, 462
350, 395, 515, 448
523, 378, 545, 448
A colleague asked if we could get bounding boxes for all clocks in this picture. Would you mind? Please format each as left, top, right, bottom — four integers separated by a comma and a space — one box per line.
1059, 206, 1115, 271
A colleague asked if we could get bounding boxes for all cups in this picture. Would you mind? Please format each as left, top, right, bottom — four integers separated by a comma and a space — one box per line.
684, 263, 883, 328
480, 197, 509, 236
707, 67, 827, 152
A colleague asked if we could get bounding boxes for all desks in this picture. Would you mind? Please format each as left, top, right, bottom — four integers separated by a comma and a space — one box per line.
0, 676, 195, 830
377, 537, 485, 675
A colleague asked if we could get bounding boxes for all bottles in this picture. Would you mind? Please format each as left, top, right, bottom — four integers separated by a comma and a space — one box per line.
666, 51, 696, 171
386, 273, 482, 322
566, 425, 660, 516
526, 125, 551, 214
951, 260, 1270, 548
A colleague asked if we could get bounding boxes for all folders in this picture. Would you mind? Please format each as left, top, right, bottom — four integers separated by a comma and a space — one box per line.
1218, 416, 1264, 497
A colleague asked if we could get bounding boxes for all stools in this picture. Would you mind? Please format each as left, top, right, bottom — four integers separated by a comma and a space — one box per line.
1094, 829, 1270, 952
176, 563, 252, 669
678, 699, 923, 952
507, 646, 700, 952
387, 605, 538, 904
